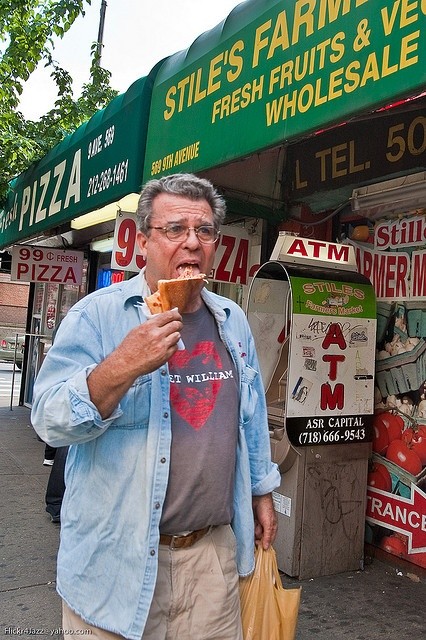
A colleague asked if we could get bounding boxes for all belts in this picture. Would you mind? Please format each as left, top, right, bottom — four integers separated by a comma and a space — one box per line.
159, 526, 216, 550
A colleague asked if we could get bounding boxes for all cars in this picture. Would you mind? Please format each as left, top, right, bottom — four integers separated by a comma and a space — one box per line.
0, 335, 24, 370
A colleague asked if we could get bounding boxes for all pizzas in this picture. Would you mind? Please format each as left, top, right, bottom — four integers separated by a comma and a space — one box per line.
144, 265, 206, 323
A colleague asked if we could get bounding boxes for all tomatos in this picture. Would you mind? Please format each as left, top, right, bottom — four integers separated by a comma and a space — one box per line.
371, 418, 389, 458
365, 462, 393, 494
401, 424, 426, 467
380, 537, 407, 560
375, 407, 404, 443
385, 439, 422, 476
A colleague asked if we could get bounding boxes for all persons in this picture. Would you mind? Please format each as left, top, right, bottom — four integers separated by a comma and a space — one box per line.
43, 445, 70, 524
28, 169, 281, 639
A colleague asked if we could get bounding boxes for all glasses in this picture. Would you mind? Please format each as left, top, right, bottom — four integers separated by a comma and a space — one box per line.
149, 224, 221, 246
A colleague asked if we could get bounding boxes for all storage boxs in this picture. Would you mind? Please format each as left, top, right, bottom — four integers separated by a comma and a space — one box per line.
368, 414, 426, 500
404, 300, 424, 339
374, 342, 426, 399
365, 470, 401, 545
377, 300, 396, 345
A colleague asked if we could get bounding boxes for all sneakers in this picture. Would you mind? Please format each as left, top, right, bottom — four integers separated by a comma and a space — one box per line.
42, 455, 54, 466
48, 511, 61, 522
